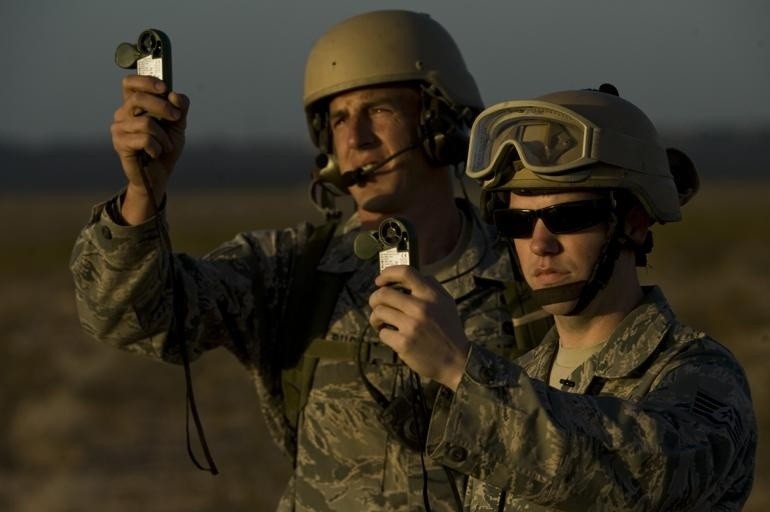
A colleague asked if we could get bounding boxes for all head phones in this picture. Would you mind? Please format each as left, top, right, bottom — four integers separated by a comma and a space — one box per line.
310, 89, 470, 197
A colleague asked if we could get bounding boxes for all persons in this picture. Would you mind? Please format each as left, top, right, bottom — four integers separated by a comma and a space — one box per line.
370, 84, 758, 511
70, 10, 555, 512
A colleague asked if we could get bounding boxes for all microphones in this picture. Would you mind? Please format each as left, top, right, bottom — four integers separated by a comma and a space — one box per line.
336, 139, 427, 189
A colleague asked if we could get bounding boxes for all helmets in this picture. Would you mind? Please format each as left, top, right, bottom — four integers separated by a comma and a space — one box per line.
299, 8, 487, 149
478, 86, 684, 227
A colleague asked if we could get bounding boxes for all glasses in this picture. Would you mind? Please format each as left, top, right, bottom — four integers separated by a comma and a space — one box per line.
491, 195, 621, 238
464, 97, 600, 179
